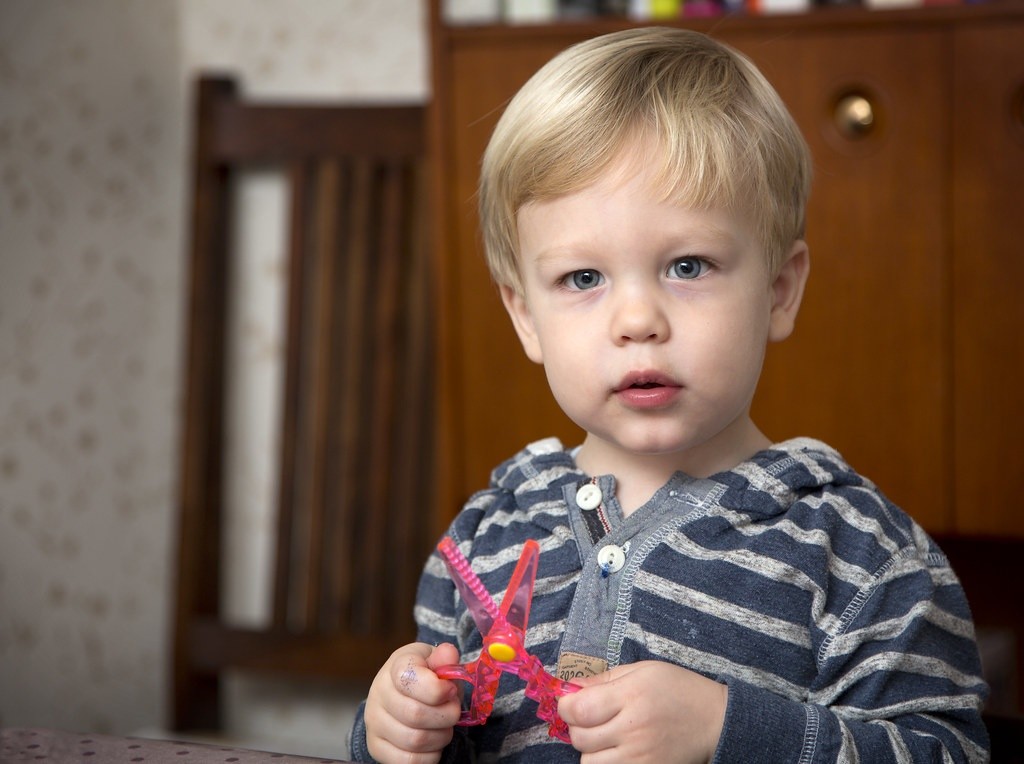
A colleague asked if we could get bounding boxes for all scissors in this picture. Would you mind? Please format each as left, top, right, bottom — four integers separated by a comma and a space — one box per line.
432, 536, 585, 747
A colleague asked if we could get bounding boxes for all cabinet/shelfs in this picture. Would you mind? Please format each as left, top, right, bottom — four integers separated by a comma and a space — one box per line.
417, 0, 1024, 563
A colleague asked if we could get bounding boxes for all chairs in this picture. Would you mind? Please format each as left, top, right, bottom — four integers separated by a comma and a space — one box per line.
127, 64, 438, 763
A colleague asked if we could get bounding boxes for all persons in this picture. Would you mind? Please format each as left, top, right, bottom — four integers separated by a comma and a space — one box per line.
345, 27, 998, 764
